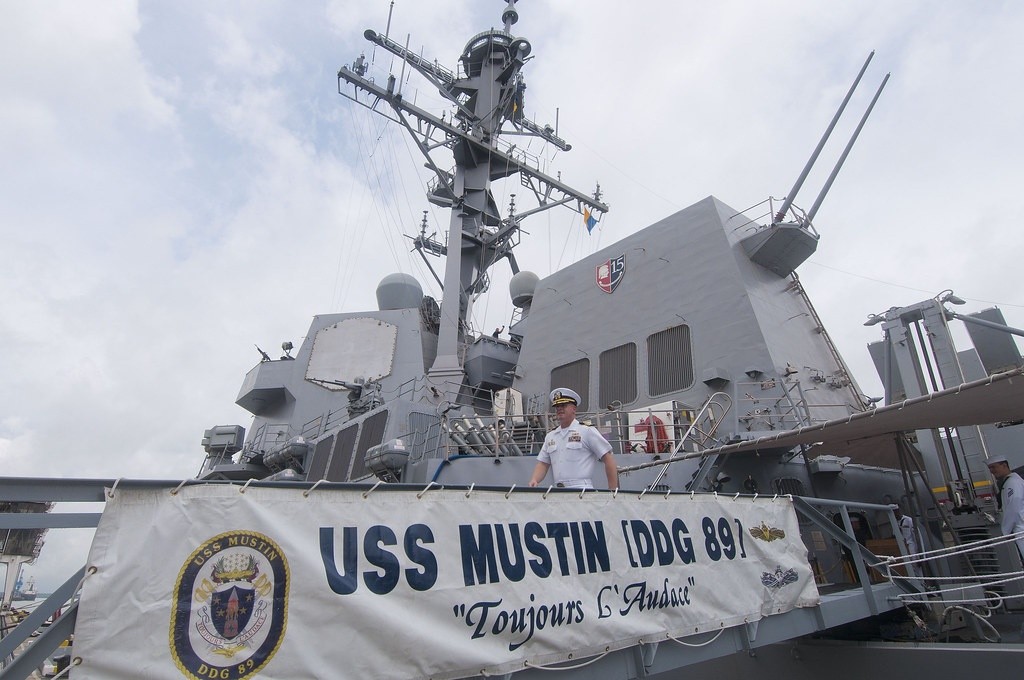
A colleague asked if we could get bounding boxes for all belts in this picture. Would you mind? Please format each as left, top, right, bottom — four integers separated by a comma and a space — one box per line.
555, 480, 591, 487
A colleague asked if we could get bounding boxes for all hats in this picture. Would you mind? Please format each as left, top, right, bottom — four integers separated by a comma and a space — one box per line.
550, 387, 581, 407
982, 454, 1006, 465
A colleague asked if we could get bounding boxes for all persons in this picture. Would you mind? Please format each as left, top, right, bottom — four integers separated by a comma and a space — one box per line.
528, 388, 620, 490
887, 504, 926, 582
982, 454, 1024, 560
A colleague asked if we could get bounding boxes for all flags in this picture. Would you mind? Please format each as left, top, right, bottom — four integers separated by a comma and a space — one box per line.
583, 208, 597, 234
